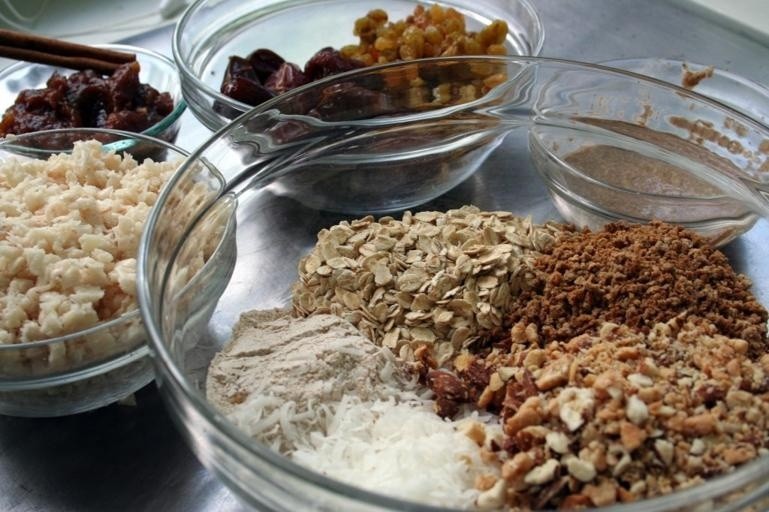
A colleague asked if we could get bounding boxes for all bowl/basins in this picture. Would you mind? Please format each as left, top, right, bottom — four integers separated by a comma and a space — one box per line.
0, 42, 189, 167
172, 2, 546, 217
0, 128, 238, 421
527, 55, 768, 253
135, 56, 768, 511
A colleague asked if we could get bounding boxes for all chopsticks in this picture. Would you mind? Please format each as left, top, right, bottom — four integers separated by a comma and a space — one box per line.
0, 28, 137, 73
